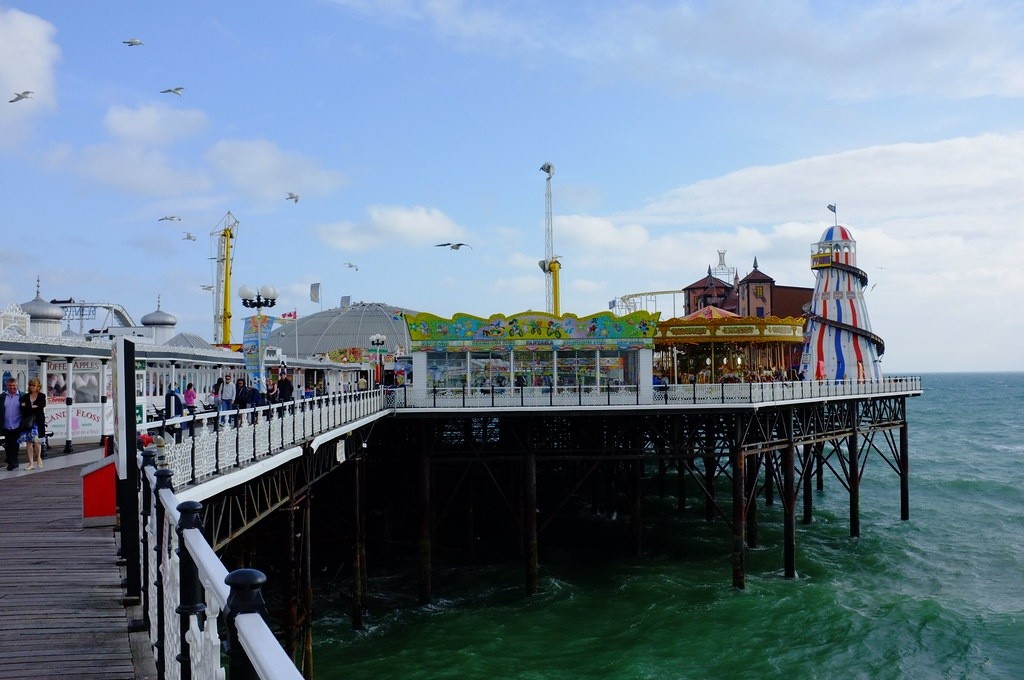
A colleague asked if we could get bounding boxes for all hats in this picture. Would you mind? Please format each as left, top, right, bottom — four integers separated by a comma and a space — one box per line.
279, 372, 285, 375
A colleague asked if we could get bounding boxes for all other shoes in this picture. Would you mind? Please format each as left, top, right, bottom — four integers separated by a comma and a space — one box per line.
37, 457, 43, 470
6, 463, 19, 471
219, 423, 225, 427
25, 466, 35, 471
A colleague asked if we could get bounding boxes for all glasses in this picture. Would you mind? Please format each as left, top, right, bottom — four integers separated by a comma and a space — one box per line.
238, 382, 243, 384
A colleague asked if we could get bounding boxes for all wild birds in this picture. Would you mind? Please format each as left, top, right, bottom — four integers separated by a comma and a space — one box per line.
159, 216, 181, 221
123, 38, 144, 47
9, 91, 33, 103
286, 192, 300, 204
160, 86, 184, 96
341, 262, 358, 271
201, 285, 213, 292
183, 232, 196, 242
435, 243, 472, 251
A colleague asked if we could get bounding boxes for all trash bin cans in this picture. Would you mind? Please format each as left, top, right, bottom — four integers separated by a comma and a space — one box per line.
80, 454, 118, 527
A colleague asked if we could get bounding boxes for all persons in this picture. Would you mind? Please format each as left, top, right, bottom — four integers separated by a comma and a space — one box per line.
166, 381, 183, 418
185, 383, 197, 415
358, 375, 367, 391
653, 369, 669, 391
212, 375, 261, 428
0, 377, 46, 471
798, 370, 805, 381
278, 372, 294, 403
267, 379, 278, 403
305, 385, 314, 399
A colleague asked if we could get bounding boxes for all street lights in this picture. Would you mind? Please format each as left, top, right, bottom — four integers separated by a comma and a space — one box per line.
369, 334, 386, 384
237, 284, 280, 393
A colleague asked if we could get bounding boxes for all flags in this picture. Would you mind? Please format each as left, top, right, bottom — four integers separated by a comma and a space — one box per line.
309, 283, 320, 303
609, 300, 614, 309
282, 311, 296, 319
827, 204, 835, 212
393, 310, 403, 320
